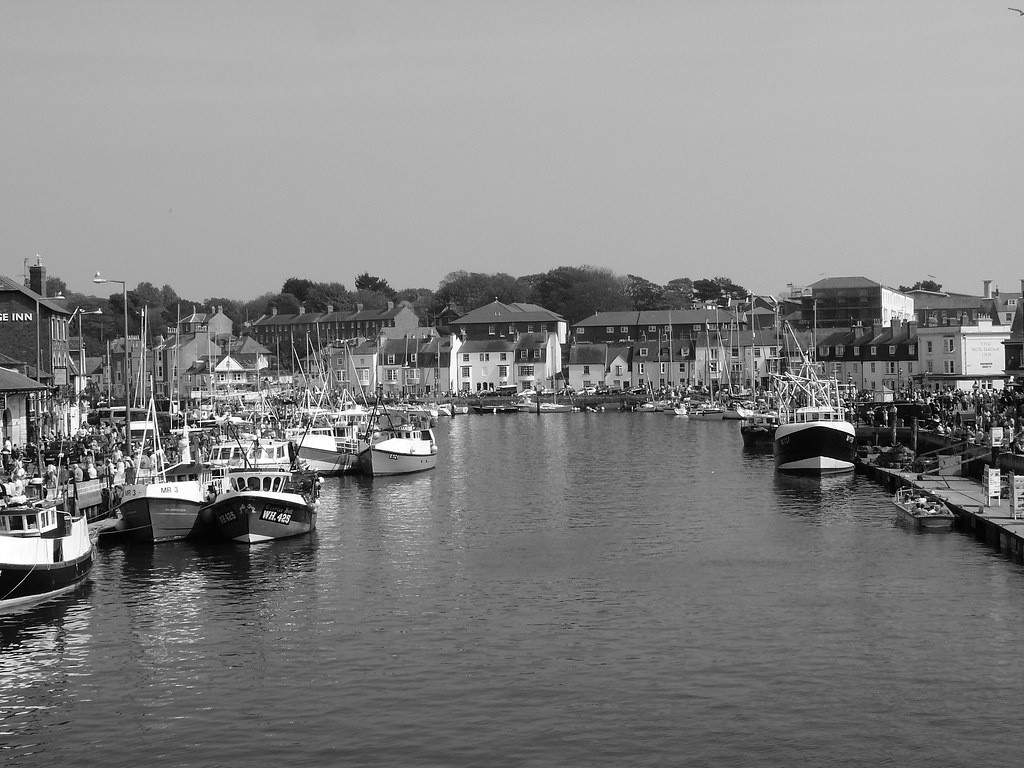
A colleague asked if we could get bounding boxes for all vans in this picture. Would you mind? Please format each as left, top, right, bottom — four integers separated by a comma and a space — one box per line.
875, 403, 932, 428
495, 385, 518, 396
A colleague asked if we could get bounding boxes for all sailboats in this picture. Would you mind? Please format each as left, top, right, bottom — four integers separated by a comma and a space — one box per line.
633, 292, 817, 443
119, 303, 468, 545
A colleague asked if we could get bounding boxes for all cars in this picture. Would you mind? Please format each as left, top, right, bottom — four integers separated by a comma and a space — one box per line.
620, 386, 644, 395
37, 441, 84, 466
593, 385, 622, 396
517, 388, 575, 396
572, 387, 596, 396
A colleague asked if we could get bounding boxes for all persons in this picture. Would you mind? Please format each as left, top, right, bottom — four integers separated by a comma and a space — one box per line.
111, 446, 123, 464
4, 437, 12, 461
356, 384, 610, 399
146, 451, 157, 464
866, 406, 875, 425
7, 477, 15, 497
640, 382, 806, 412
0, 382, 345, 500
74, 425, 90, 449
80, 466, 90, 481
12, 475, 22, 495
918, 493, 927, 502
96, 461, 104, 479
839, 396, 846, 408
880, 407, 888, 428
73, 464, 83, 482
902, 492, 936, 515
48, 461, 57, 475
87, 464, 97, 479
829, 375, 1024, 454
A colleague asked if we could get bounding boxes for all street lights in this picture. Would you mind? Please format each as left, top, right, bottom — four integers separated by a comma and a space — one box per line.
37, 296, 66, 436
93, 279, 132, 456
847, 372, 853, 425
972, 380, 979, 417
79, 311, 103, 428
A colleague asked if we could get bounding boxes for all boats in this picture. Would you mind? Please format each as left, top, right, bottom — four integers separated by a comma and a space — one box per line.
891, 486, 955, 527
471, 397, 575, 413
771, 299, 857, 476
0, 397, 99, 607
